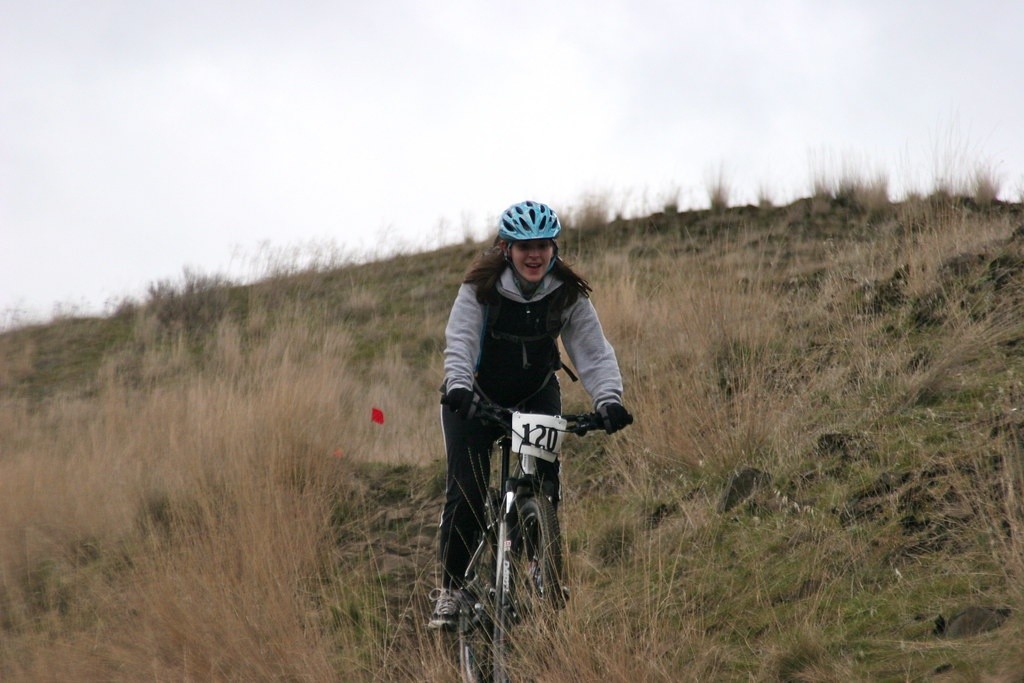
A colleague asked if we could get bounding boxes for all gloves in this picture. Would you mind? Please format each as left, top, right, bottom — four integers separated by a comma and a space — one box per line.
597, 402, 630, 434
446, 388, 480, 420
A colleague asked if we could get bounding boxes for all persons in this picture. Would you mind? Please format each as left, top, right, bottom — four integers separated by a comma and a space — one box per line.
424, 201, 631, 631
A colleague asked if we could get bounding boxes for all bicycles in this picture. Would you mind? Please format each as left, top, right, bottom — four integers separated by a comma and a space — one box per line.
435, 388, 635, 683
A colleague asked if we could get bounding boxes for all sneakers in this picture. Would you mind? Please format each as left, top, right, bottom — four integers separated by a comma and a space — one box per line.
428, 587, 462, 632
536, 575, 569, 603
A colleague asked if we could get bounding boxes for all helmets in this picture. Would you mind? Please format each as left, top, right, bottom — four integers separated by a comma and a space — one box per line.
498, 201, 561, 241
